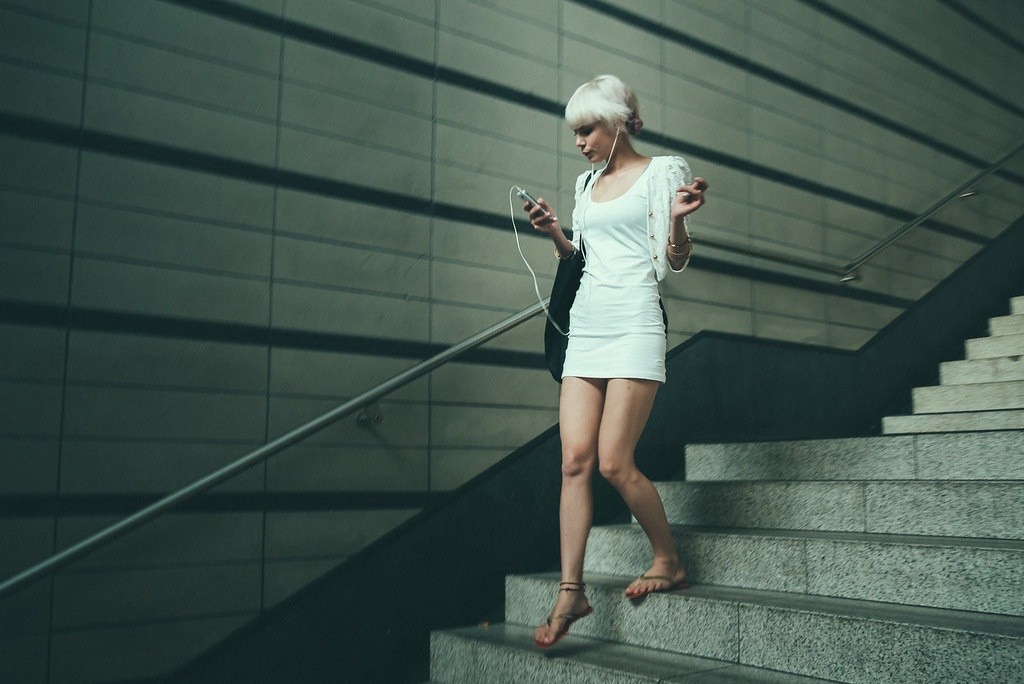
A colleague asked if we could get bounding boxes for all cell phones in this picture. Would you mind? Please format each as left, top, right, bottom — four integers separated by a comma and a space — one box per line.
516, 189, 553, 226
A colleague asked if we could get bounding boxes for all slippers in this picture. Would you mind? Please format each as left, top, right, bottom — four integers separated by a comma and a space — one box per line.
535, 606, 592, 648
625, 568, 690, 599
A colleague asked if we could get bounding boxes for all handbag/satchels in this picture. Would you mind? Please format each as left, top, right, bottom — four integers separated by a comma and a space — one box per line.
544, 250, 669, 384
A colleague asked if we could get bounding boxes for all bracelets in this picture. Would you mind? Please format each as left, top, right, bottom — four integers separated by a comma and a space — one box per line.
668, 257, 685, 262
555, 243, 576, 262
667, 248, 690, 255
668, 233, 689, 248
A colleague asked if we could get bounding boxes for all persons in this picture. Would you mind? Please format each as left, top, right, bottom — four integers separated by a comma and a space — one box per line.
523, 74, 708, 648
